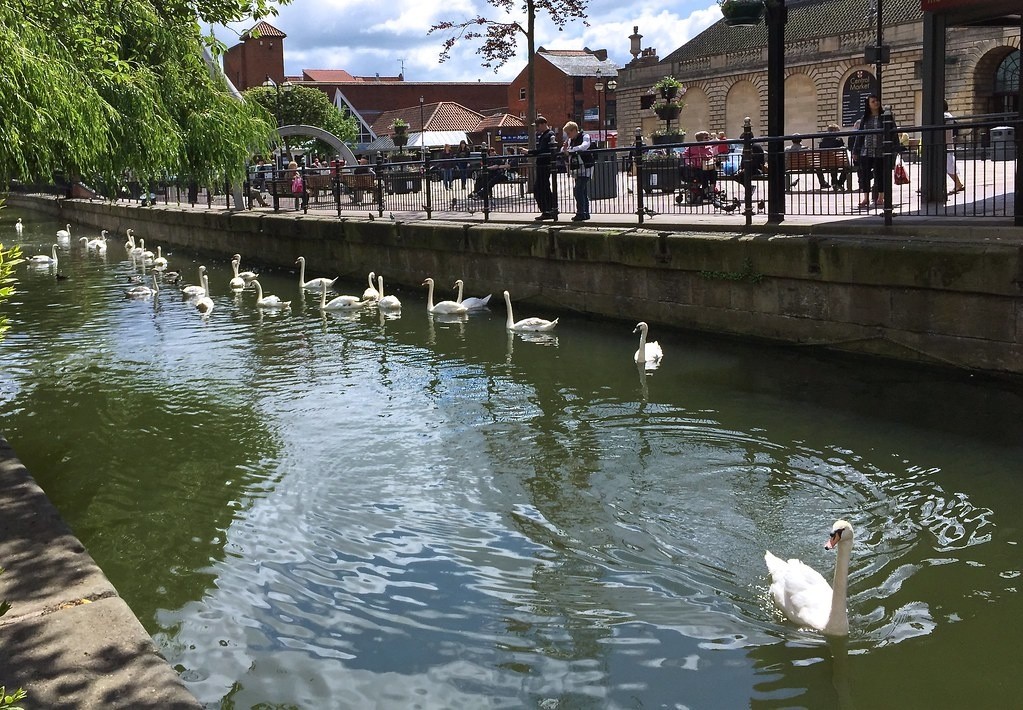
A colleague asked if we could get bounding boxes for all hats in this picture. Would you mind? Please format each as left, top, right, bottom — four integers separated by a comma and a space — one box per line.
358, 158, 368, 165
531, 117, 547, 126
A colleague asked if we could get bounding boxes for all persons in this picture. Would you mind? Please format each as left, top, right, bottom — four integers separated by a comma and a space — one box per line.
518, 116, 558, 222
915, 99, 965, 196
558, 120, 595, 221
818, 123, 849, 191
892, 132, 910, 170
467, 147, 518, 200
679, 131, 719, 196
847, 119, 861, 192
455, 139, 470, 190
784, 133, 831, 188
252, 160, 266, 192
625, 135, 649, 174
248, 186, 271, 207
440, 144, 455, 190
733, 132, 765, 195
852, 95, 900, 205
706, 131, 729, 158
283, 156, 375, 211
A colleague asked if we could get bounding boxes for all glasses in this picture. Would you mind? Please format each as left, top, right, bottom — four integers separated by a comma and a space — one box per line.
704, 136, 710, 139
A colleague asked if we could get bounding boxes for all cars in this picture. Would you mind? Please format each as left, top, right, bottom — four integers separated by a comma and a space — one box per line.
428, 151, 503, 182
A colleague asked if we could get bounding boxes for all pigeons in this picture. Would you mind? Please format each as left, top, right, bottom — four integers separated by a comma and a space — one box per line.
711, 196, 765, 215
642, 206, 662, 220
675, 190, 684, 204
626, 186, 634, 195
261, 190, 494, 221
789, 177, 801, 188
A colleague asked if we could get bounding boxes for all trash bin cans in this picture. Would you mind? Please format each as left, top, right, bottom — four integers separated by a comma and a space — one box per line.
584, 149, 618, 200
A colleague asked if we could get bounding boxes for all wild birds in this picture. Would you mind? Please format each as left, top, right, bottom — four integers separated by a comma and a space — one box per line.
54, 273, 70, 280
163, 269, 183, 278
125, 275, 142, 283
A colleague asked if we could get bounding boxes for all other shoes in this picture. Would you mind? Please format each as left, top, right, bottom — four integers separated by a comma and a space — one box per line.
949, 183, 965, 193
751, 186, 756, 195
858, 199, 869, 205
571, 212, 590, 221
489, 195, 493, 200
832, 184, 838, 191
262, 203, 270, 207
821, 183, 831, 189
468, 193, 474, 198
535, 213, 552, 220
869, 200, 875, 207
915, 188, 921, 193
473, 193, 479, 199
876, 198, 884, 205
834, 181, 845, 190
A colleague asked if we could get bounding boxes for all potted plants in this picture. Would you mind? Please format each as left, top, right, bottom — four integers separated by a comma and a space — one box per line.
642, 75, 684, 165
716, 0, 765, 28
384, 119, 421, 177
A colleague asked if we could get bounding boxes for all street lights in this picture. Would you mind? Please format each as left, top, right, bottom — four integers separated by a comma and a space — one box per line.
418, 95, 425, 144
262, 72, 283, 169
593, 66, 604, 142
595, 77, 619, 140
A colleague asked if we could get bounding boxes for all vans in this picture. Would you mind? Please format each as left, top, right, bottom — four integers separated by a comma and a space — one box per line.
249, 164, 279, 179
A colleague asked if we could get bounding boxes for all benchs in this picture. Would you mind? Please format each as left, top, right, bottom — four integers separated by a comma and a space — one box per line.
717, 147, 857, 194
222, 174, 385, 204
489, 167, 529, 199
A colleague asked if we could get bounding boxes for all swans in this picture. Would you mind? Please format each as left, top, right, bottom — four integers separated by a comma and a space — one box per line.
25, 243, 62, 263
762, 516, 856, 637
14, 217, 24, 228
362, 271, 380, 300
56, 223, 72, 237
421, 277, 469, 315
375, 275, 402, 309
452, 279, 493, 308
181, 264, 215, 314
228, 253, 260, 291
78, 229, 109, 248
249, 279, 292, 306
503, 289, 560, 332
295, 256, 339, 288
318, 279, 371, 310
122, 267, 161, 298
124, 227, 168, 267
631, 320, 664, 363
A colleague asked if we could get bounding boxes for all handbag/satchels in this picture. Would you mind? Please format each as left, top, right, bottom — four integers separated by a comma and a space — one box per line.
894, 164, 910, 185
760, 161, 768, 174
895, 154, 906, 173
703, 158, 714, 171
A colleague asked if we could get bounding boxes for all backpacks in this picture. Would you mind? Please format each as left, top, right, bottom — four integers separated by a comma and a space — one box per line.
292, 177, 303, 194
